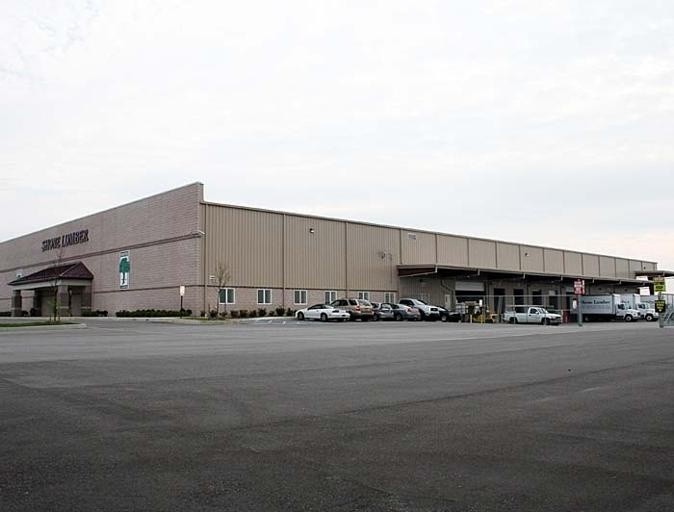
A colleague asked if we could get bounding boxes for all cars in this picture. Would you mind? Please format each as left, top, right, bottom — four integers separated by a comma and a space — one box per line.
295, 297, 460, 321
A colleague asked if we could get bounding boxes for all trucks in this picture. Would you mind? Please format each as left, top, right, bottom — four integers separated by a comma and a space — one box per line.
569, 293, 659, 322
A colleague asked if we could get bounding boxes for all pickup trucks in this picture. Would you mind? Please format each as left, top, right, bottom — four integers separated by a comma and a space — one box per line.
502, 306, 561, 325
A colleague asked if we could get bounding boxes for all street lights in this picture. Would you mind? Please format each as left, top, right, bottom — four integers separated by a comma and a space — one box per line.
179, 284, 185, 318
